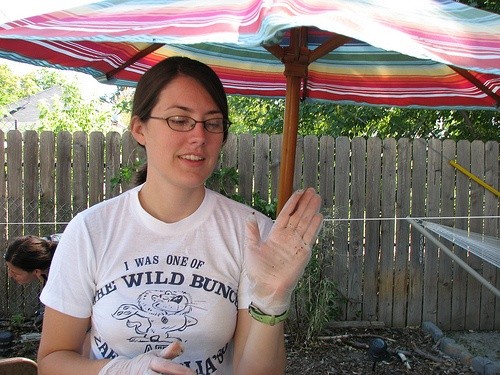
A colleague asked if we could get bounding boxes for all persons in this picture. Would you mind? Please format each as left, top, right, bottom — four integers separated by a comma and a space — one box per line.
5, 234, 63, 287
38, 55, 323, 375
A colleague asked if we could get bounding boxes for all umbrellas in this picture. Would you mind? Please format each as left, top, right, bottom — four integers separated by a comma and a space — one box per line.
1, 0, 500, 220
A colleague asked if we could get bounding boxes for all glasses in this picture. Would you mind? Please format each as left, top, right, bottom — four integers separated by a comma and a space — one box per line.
144, 114, 233, 133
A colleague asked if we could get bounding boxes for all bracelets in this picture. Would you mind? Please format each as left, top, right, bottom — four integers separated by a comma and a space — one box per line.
247, 301, 290, 324
